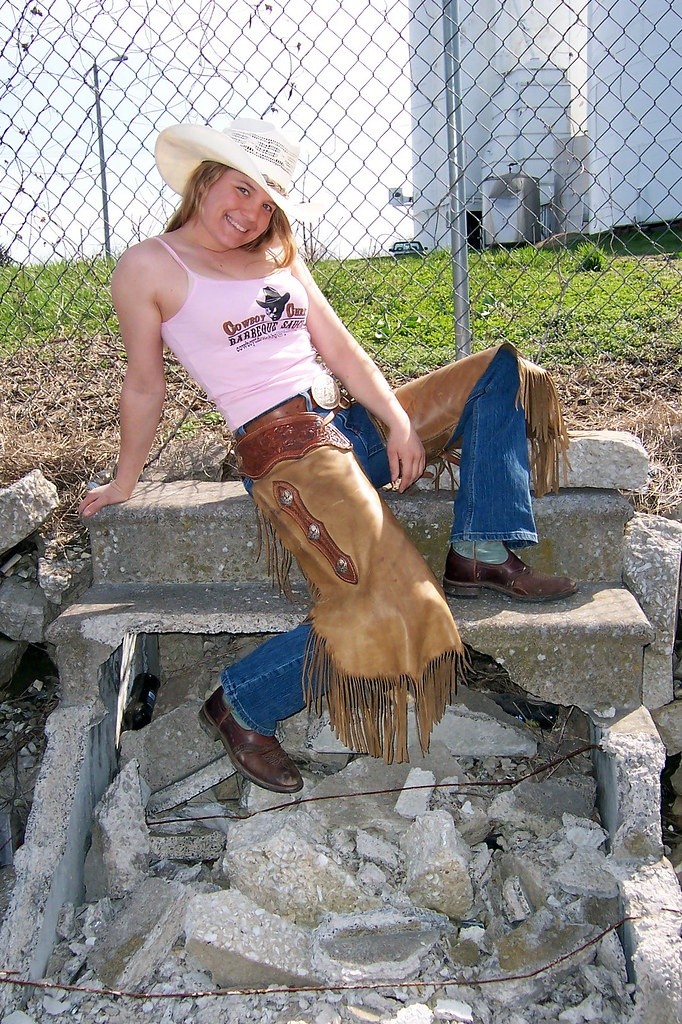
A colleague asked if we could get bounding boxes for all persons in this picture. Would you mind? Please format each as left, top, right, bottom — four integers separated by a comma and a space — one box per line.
78, 116, 580, 795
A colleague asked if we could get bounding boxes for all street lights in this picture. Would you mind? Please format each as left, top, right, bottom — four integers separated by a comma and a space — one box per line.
93, 55, 130, 261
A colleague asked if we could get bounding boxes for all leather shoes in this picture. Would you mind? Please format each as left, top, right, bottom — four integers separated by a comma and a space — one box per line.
443, 541, 579, 602
198, 684, 304, 793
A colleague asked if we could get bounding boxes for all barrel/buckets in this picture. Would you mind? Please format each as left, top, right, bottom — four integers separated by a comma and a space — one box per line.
490, 54, 579, 241
480, 161, 544, 247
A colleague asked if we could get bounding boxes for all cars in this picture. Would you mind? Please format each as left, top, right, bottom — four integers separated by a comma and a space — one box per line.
390, 240, 429, 258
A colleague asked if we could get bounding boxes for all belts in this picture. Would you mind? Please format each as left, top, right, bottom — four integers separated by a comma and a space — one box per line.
322, 393, 352, 425
244, 375, 339, 435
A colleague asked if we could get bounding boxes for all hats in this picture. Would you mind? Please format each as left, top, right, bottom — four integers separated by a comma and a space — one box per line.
154, 115, 310, 222
